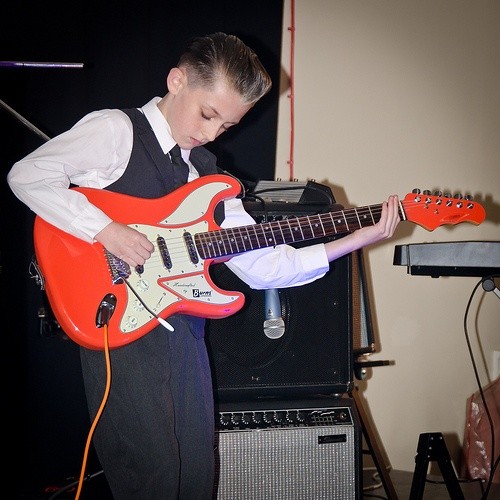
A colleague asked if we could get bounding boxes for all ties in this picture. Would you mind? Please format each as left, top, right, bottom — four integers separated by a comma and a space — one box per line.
169, 143, 190, 190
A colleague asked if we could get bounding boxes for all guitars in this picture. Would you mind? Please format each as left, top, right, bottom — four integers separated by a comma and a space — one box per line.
29, 175, 486, 350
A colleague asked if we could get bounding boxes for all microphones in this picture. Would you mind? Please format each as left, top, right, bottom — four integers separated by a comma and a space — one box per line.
262, 290, 284, 342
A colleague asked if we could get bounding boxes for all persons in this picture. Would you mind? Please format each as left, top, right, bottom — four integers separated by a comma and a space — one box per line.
5, 31, 402, 499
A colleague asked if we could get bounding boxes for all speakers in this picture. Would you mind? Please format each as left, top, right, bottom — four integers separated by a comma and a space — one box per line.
211, 397, 360, 500
198, 200, 351, 395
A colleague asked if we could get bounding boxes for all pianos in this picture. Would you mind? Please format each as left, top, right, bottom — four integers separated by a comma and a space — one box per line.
393, 241, 500, 278
